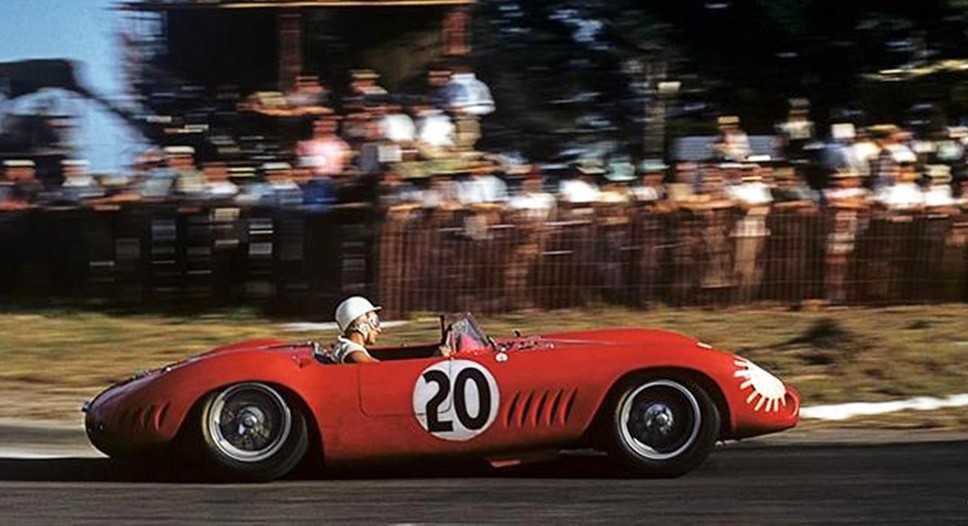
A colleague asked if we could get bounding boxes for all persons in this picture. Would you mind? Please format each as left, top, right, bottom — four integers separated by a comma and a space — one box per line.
1, 59, 968, 312
329, 296, 383, 364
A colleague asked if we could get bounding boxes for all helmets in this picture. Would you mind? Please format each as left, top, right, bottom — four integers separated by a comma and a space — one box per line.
335, 296, 383, 331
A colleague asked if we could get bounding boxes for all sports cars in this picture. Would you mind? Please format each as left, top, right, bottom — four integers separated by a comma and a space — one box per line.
81, 314, 799, 485
